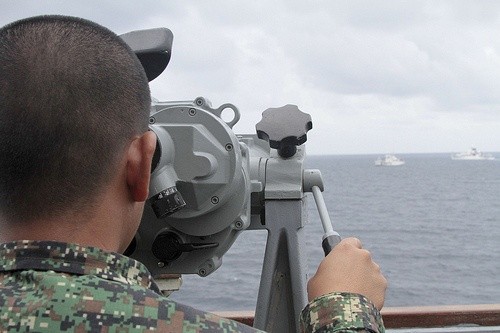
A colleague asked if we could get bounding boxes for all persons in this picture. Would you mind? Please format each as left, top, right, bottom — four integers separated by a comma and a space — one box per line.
0, 15, 389, 333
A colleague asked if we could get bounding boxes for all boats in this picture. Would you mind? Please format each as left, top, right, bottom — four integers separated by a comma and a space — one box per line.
373, 154, 406, 168
450, 146, 492, 160
